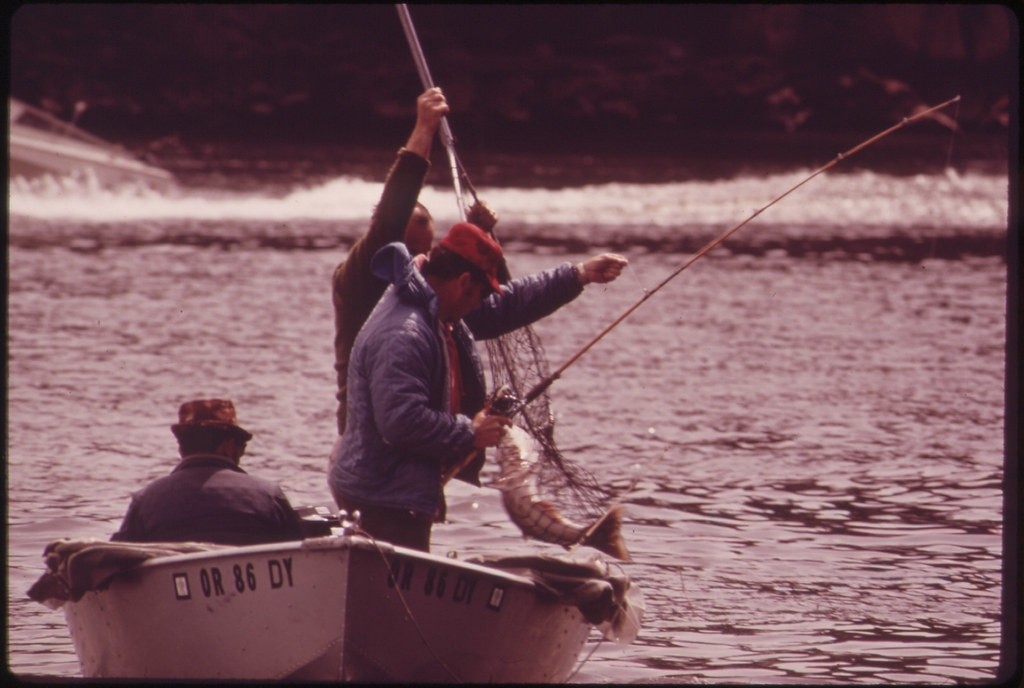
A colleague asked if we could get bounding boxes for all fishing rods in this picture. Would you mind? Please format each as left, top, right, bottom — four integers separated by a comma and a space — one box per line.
438, 90, 964, 491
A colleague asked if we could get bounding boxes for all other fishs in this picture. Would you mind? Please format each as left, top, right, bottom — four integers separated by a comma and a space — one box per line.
457, 548, 632, 635
486, 423, 632, 562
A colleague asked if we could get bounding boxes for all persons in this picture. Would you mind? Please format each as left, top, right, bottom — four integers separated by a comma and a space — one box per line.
332, 86, 499, 437
327, 222, 629, 555
108, 398, 305, 547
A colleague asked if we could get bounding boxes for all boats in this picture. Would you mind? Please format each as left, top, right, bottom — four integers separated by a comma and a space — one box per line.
7, 98, 182, 198
340, 511, 614, 687
42, 507, 346, 686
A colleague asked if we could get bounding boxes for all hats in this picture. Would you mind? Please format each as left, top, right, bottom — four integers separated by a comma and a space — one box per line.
170, 398, 253, 442
440, 222, 507, 296
